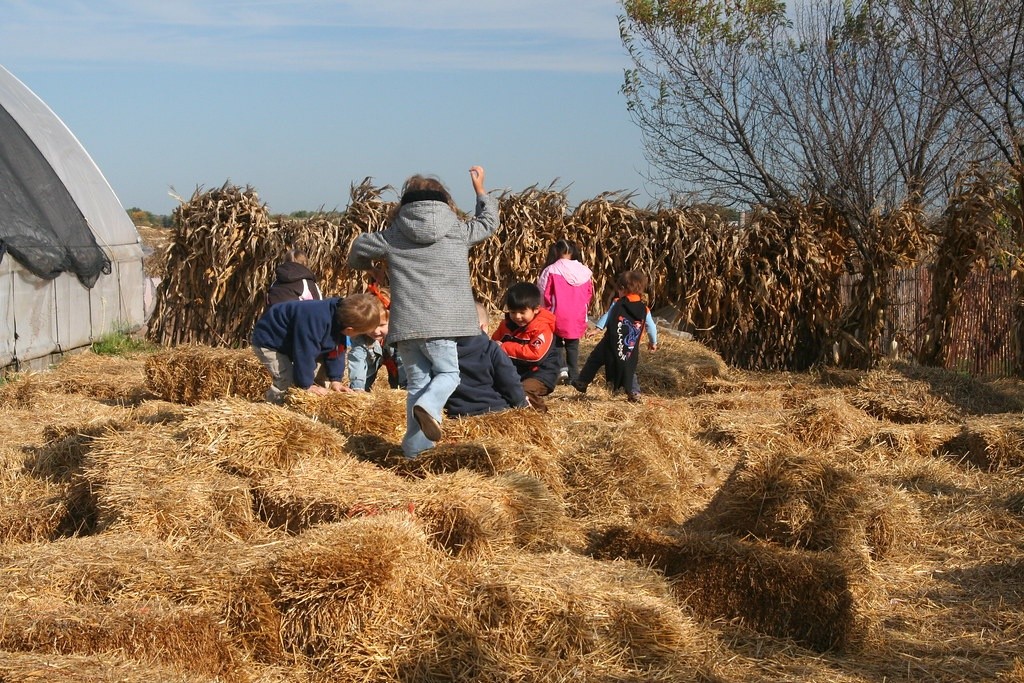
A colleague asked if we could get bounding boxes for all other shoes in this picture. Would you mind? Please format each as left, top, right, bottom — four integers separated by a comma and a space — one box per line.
414, 407, 441, 441
628, 393, 643, 403
572, 379, 588, 392
556, 370, 569, 385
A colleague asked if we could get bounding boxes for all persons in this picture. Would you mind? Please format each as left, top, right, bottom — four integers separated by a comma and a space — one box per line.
349, 166, 500, 458
252, 293, 380, 407
491, 282, 560, 415
535, 239, 595, 386
347, 285, 407, 393
445, 300, 531, 421
572, 271, 657, 403
268, 249, 323, 303
